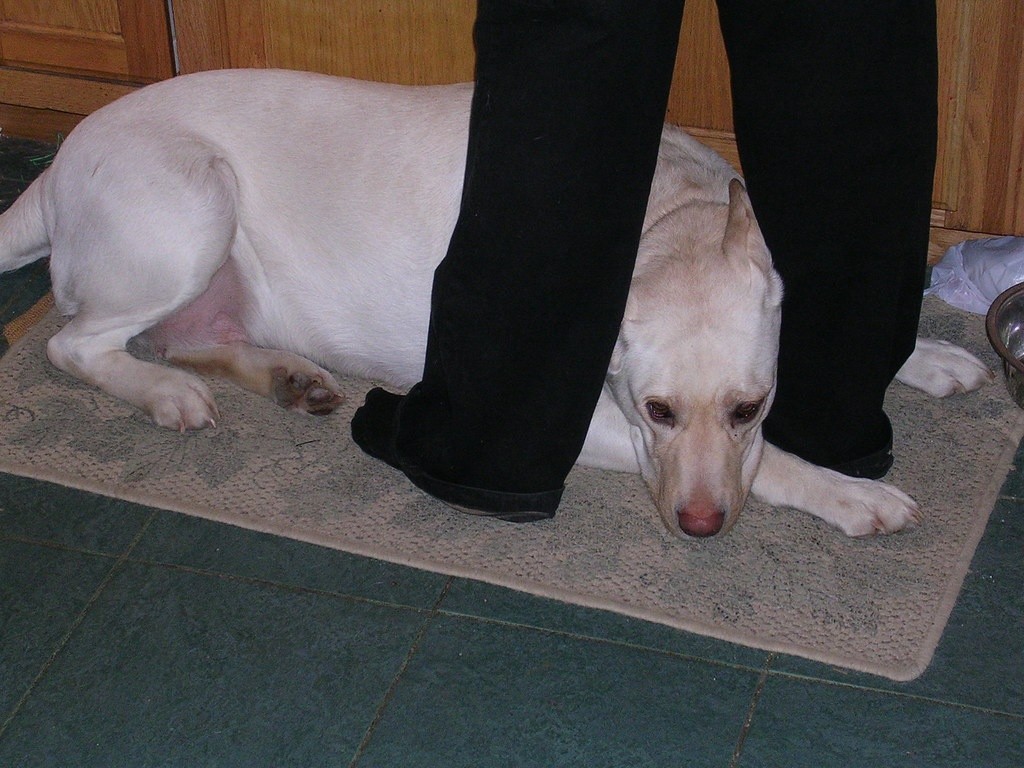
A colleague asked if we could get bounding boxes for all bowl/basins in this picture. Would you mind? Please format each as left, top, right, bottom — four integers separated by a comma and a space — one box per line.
984, 279, 1024, 409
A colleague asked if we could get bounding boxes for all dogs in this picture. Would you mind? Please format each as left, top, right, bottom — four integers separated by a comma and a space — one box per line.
0, 69, 995, 544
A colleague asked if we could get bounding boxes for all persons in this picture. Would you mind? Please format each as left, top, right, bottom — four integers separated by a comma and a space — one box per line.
352, 0, 937, 523
